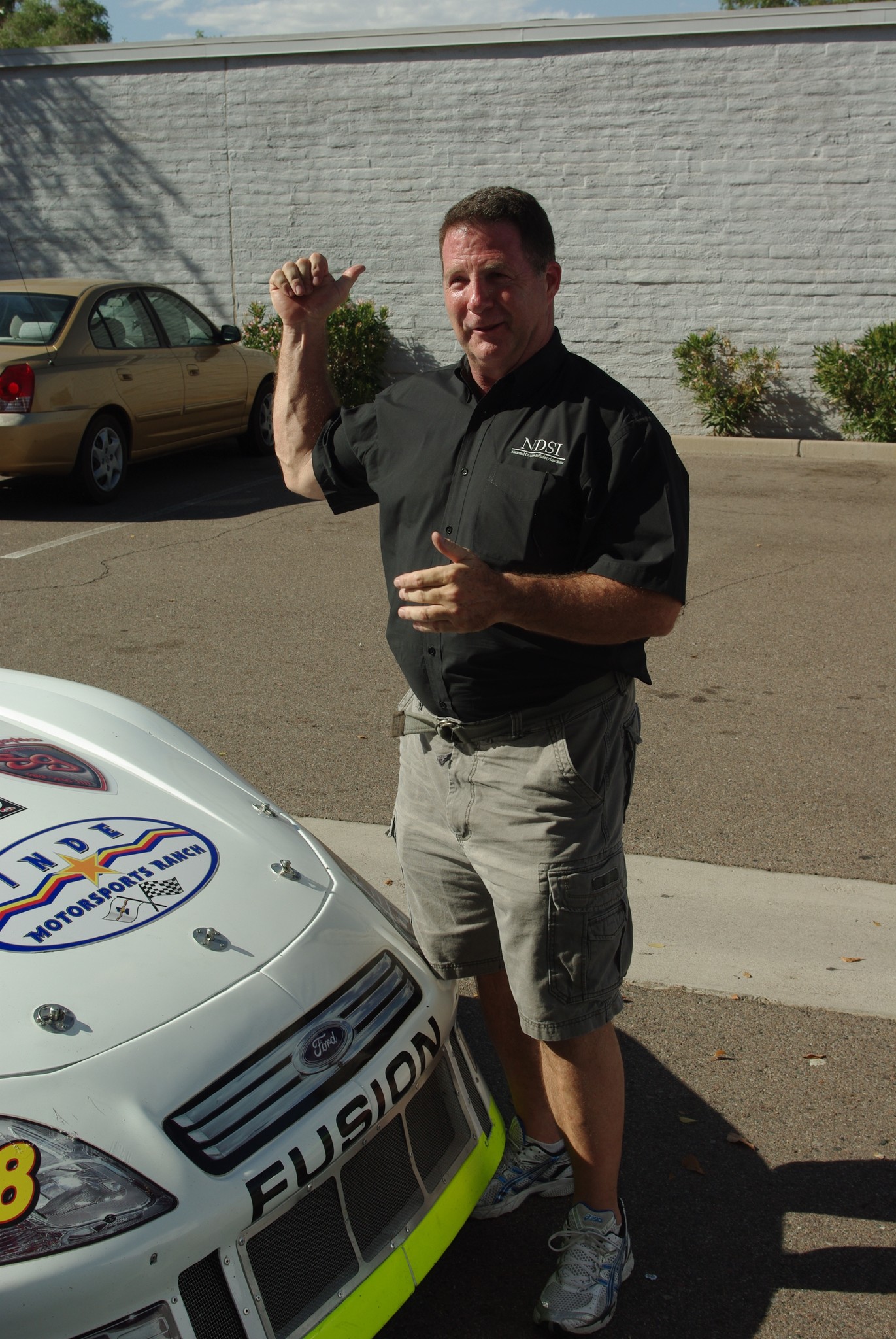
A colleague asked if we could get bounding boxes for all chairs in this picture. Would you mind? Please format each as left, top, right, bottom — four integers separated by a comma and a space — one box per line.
90, 318, 136, 347
19, 321, 57, 343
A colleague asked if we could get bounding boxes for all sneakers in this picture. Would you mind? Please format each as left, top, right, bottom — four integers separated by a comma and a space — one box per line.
470, 1116, 576, 1219
534, 1197, 634, 1334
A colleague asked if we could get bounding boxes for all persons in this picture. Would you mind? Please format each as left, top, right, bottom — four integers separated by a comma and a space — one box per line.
269, 185, 690, 1339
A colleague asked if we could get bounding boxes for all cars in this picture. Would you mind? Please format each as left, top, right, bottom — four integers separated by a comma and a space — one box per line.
0, 667, 507, 1339
0, 275, 278, 502
